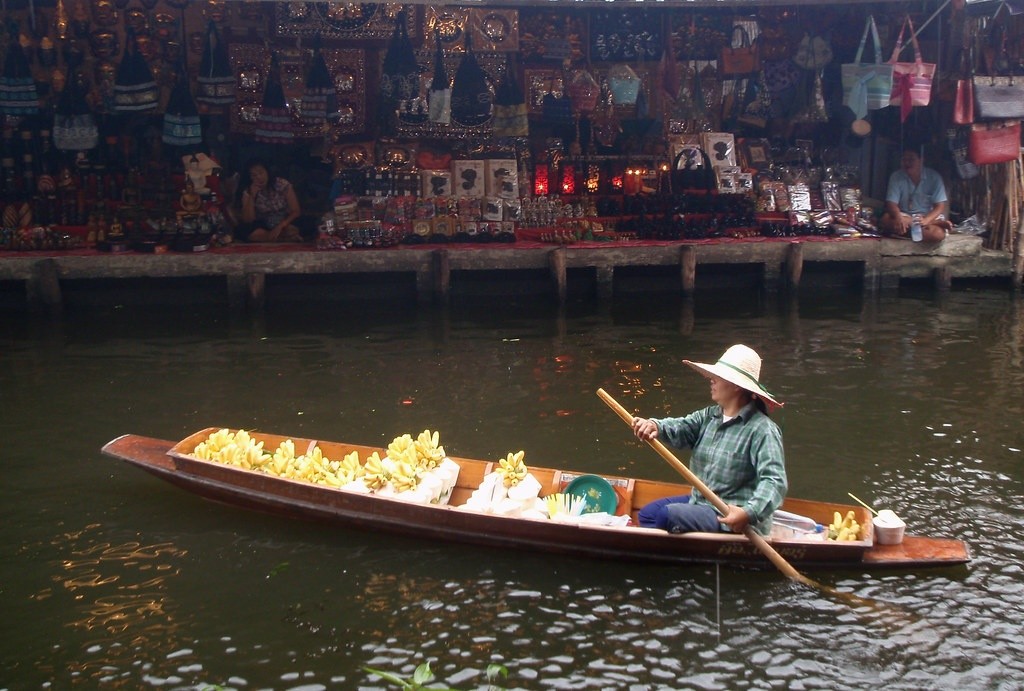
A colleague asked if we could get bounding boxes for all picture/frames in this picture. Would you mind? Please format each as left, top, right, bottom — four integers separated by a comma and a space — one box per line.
412, 217, 455, 238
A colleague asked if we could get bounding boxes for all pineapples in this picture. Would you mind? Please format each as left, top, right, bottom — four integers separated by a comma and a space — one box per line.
2, 186, 18, 228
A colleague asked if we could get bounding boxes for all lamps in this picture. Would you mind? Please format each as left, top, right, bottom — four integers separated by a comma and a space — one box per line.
533, 161, 550, 195
584, 162, 600, 195
557, 162, 575, 195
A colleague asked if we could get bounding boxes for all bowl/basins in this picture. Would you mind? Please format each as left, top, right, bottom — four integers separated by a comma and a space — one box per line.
563, 474, 617, 516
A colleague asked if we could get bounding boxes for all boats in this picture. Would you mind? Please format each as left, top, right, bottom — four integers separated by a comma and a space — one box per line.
102, 425, 972, 573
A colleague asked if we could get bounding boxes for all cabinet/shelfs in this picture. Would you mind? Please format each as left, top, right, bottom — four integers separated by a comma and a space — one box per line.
742, 137, 771, 168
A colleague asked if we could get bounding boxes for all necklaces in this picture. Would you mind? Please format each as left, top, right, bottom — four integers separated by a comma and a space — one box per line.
907, 179, 920, 211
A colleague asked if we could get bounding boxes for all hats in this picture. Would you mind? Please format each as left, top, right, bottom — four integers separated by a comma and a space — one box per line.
682, 344, 783, 410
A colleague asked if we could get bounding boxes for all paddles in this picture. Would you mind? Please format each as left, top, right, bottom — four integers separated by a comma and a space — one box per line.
596, 388, 817, 585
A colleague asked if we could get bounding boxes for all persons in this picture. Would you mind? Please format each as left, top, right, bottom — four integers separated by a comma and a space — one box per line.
239, 158, 303, 242
631, 344, 788, 534
880, 146, 952, 241
86, 211, 123, 241
0, 0, 232, 218
177, 158, 211, 227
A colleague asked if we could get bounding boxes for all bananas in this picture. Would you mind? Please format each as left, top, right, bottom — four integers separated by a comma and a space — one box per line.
827, 509, 860, 542
494, 450, 527, 488
187, 430, 446, 497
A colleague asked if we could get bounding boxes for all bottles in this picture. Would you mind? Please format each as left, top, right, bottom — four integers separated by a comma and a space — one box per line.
771, 509, 823, 533
911, 214, 922, 242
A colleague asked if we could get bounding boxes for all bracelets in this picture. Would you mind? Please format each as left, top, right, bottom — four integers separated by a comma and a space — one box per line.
281, 220, 288, 228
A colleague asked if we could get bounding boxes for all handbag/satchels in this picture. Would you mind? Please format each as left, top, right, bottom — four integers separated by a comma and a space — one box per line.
0, 0, 1024, 199
